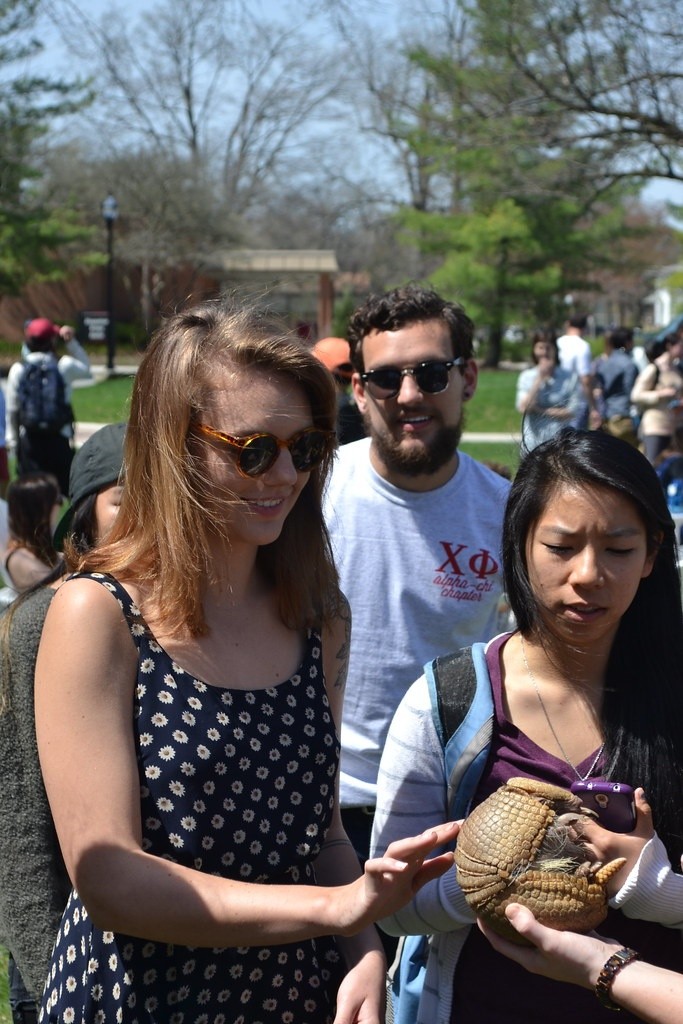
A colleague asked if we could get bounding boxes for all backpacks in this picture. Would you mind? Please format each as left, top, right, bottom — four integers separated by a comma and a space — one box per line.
629, 357, 662, 427
16, 355, 75, 437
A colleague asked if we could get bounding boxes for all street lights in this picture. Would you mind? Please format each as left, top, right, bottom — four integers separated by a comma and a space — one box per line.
98, 190, 122, 369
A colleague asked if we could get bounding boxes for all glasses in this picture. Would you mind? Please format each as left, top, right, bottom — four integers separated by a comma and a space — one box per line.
360, 359, 461, 394
190, 421, 329, 476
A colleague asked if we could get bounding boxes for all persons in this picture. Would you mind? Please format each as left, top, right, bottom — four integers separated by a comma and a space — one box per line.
365, 424, 683, 1024
4, 316, 92, 501
32, 297, 463, 1023
1, 471, 64, 594
513, 302, 682, 518
0, 361, 12, 498
0, 421, 127, 1024
309, 335, 369, 447
474, 901, 683, 1023
319, 285, 513, 972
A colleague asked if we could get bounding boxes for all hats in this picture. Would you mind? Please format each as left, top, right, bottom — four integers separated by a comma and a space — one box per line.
24, 318, 60, 351
50, 422, 127, 553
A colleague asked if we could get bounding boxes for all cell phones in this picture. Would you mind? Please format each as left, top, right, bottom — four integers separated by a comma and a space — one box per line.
571, 780, 637, 834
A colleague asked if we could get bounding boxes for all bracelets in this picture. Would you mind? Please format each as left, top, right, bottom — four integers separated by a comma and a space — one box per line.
595, 947, 641, 1015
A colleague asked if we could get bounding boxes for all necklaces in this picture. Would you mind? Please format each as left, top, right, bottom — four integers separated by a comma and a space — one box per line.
519, 628, 606, 783
